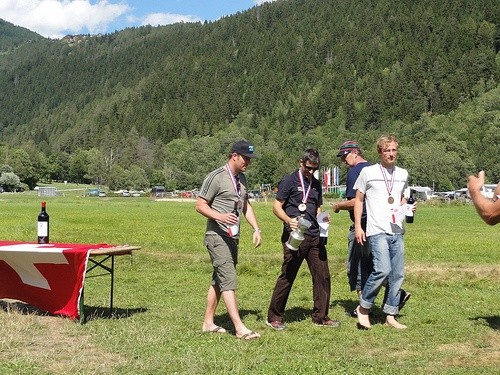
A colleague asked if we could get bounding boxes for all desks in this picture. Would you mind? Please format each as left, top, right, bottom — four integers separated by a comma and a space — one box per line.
0, 240, 142, 325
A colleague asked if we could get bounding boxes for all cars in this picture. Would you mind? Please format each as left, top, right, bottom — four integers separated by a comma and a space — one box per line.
123, 191, 130, 197
132, 191, 140, 197
172, 188, 201, 198
98, 191, 107, 197
403, 184, 498, 201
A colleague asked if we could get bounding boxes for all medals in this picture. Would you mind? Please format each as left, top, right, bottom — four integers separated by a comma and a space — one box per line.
238, 201, 242, 210
298, 204, 307, 212
388, 197, 394, 204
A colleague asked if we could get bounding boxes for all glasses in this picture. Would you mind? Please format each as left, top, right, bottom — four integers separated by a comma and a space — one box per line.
304, 165, 317, 171
233, 176, 241, 192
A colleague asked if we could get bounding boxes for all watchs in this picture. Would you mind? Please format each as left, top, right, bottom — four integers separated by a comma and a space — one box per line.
254, 228, 262, 233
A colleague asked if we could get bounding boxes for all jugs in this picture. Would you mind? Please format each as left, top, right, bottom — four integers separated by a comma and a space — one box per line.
285, 216, 312, 251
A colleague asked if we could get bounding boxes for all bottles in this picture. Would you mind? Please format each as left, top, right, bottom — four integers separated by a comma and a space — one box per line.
228, 202, 240, 238
319, 226, 328, 245
406, 196, 414, 223
37, 201, 49, 244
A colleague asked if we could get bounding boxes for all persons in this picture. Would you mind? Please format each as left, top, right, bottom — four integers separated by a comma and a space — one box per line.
353, 136, 418, 329
265, 149, 341, 331
468, 170, 500, 226
331, 141, 411, 313
194, 140, 263, 340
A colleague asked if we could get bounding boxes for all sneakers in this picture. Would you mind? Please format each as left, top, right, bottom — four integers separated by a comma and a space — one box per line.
399, 290, 411, 311
266, 319, 285, 330
350, 307, 373, 318
312, 318, 341, 327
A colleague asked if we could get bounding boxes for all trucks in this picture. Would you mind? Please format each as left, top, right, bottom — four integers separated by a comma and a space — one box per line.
153, 185, 166, 197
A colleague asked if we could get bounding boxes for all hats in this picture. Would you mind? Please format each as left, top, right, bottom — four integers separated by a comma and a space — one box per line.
231, 140, 257, 158
336, 141, 360, 157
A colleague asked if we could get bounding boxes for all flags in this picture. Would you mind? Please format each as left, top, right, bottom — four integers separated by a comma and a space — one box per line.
323, 167, 339, 187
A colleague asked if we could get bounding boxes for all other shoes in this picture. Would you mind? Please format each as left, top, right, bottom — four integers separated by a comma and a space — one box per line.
355, 305, 371, 329
387, 317, 407, 330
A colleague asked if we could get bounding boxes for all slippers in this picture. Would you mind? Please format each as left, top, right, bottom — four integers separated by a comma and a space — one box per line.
237, 332, 261, 339
203, 327, 226, 333
